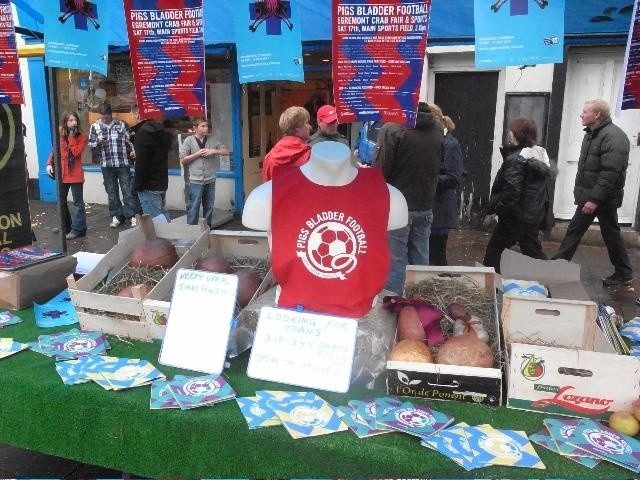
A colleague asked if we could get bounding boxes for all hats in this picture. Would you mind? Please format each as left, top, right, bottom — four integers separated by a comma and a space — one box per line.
317, 105, 337, 125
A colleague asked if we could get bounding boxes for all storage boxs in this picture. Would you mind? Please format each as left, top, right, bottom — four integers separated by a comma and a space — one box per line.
0, 255, 78, 311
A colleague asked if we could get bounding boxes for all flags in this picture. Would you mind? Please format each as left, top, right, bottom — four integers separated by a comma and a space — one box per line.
43, 0, 111, 79
613, 0, 640, 109
232, 0, 307, 85
0, 0, 28, 106
331, 0, 432, 129
473, 0, 565, 70
122, 0, 209, 120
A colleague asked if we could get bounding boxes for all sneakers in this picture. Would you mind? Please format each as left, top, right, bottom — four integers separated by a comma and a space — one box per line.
130, 217, 137, 227
110, 216, 121, 227
603, 273, 634, 287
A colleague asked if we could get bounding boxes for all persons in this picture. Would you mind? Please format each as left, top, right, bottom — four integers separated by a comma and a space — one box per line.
482, 118, 556, 277
243, 141, 410, 315
261, 106, 350, 182
551, 96, 638, 289
45, 102, 229, 239
355, 102, 464, 297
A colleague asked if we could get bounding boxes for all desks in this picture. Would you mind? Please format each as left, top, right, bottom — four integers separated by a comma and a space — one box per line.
0, 299, 639, 479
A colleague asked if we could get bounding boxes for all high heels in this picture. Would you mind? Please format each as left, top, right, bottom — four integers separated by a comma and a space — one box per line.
65, 229, 87, 239
52, 226, 72, 234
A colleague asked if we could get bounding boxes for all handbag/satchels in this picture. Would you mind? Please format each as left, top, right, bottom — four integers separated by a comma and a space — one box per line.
49, 162, 55, 180
540, 199, 556, 230
